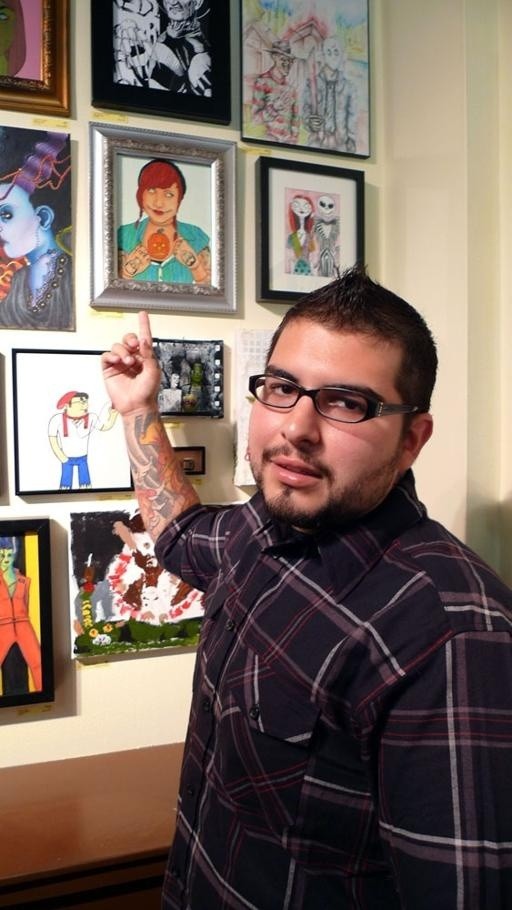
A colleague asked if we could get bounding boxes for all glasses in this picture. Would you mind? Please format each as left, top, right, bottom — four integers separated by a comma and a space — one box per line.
249, 373, 425, 423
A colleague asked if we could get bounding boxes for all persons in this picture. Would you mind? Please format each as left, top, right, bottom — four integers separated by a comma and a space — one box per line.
101, 261, 512, 910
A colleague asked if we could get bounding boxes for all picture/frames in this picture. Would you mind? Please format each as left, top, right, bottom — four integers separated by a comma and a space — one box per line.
0, 516, 55, 707
238, 0, 371, 159
88, 120, 238, 315
90, 0, 232, 126
0, 0, 72, 118
254, 154, 366, 305
11, 347, 135, 497
152, 338, 225, 420
